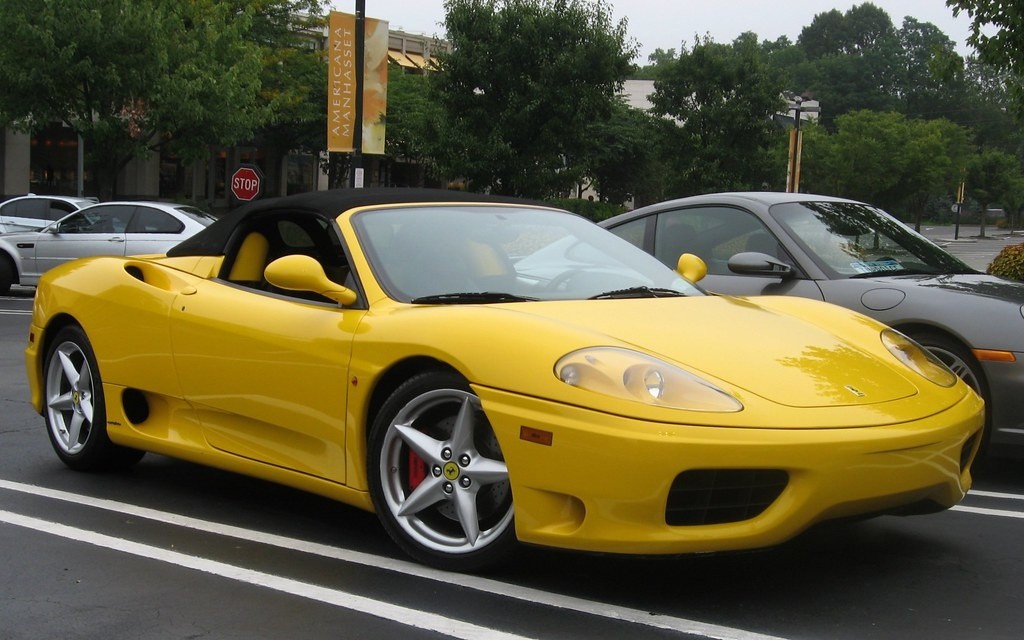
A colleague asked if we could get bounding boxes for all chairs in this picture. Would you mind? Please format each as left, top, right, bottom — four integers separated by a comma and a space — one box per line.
656, 221, 701, 270
746, 235, 781, 272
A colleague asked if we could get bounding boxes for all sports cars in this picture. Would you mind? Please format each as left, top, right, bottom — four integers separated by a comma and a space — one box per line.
22, 184, 989, 575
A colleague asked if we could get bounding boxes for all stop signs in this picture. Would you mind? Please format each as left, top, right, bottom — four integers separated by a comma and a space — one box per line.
232, 168, 260, 201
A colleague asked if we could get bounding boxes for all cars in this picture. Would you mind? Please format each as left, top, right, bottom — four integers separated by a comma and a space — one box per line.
0, 200, 219, 297
514, 191, 1023, 483
0, 196, 156, 235
